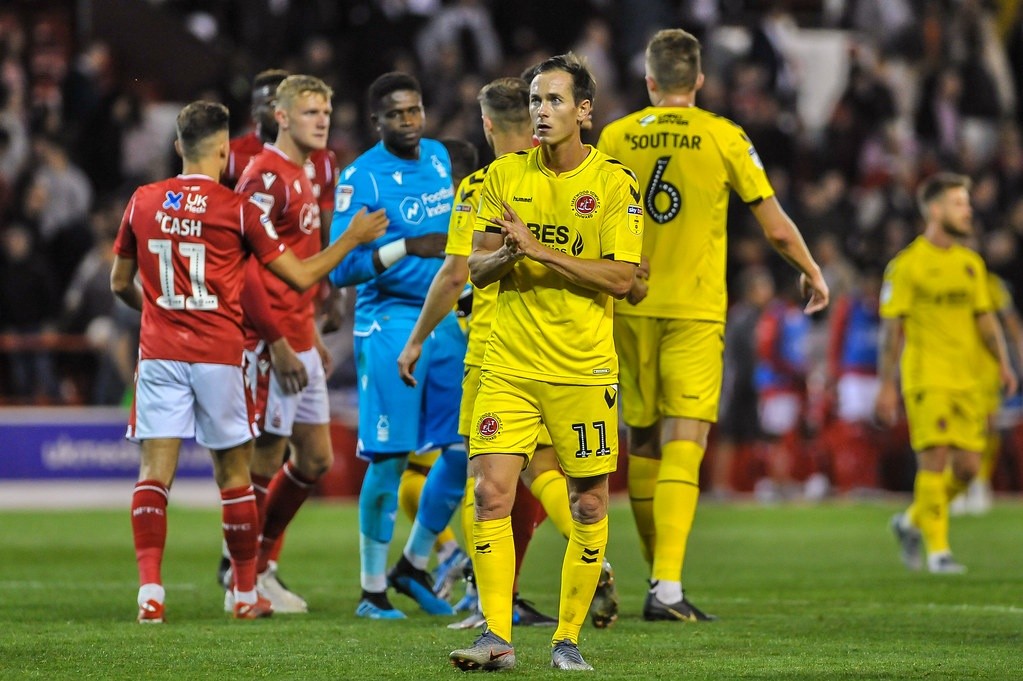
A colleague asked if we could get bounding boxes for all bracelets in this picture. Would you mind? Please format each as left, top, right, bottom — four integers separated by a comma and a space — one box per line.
379, 237, 407, 270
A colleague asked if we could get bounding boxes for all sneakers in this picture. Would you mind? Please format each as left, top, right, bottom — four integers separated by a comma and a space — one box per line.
212, 539, 310, 619
445, 613, 488, 632
928, 556, 969, 577
548, 642, 594, 674
510, 596, 561, 628
589, 557, 618, 630
891, 520, 924, 571
450, 568, 480, 613
446, 628, 518, 674
431, 547, 474, 599
356, 595, 408, 618
136, 601, 166, 624
389, 552, 454, 615
645, 589, 715, 625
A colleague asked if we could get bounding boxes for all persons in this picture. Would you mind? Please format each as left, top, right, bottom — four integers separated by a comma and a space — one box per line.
328, 73, 469, 617
111, 100, 390, 623
446, 51, 645, 670
594, 28, 829, 624
1, 1, 1022, 631
222, 76, 335, 615
873, 171, 1018, 576
